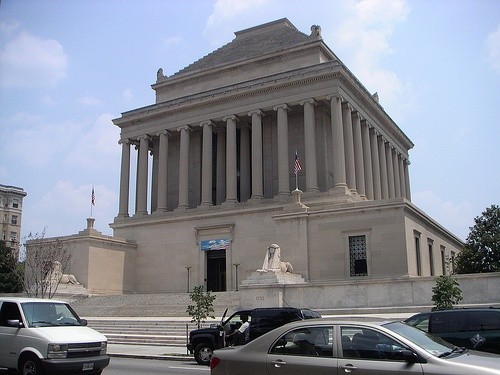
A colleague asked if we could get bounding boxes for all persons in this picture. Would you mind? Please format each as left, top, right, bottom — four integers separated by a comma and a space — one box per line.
226, 314, 250, 346
359, 329, 406, 365
39, 304, 67, 326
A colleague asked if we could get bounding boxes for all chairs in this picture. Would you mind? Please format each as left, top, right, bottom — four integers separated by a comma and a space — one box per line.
338, 335, 361, 357
352, 331, 371, 358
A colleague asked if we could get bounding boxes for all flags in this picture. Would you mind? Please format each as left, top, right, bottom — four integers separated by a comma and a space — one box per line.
294, 153, 301, 174
91, 187, 95, 205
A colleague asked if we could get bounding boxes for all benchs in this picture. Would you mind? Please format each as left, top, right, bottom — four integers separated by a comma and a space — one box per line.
272, 333, 320, 356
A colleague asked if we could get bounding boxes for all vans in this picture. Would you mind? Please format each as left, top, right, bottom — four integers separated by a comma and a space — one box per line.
404, 308, 500, 355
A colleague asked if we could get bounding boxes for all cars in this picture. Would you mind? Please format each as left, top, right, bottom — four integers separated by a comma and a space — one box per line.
210, 317, 500, 374
0, 296, 111, 375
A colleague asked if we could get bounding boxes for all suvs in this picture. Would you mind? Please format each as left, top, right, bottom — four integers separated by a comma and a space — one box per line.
186, 306, 329, 365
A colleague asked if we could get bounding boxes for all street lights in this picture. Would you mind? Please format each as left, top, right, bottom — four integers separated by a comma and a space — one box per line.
233, 263, 240, 291
185, 264, 192, 293
